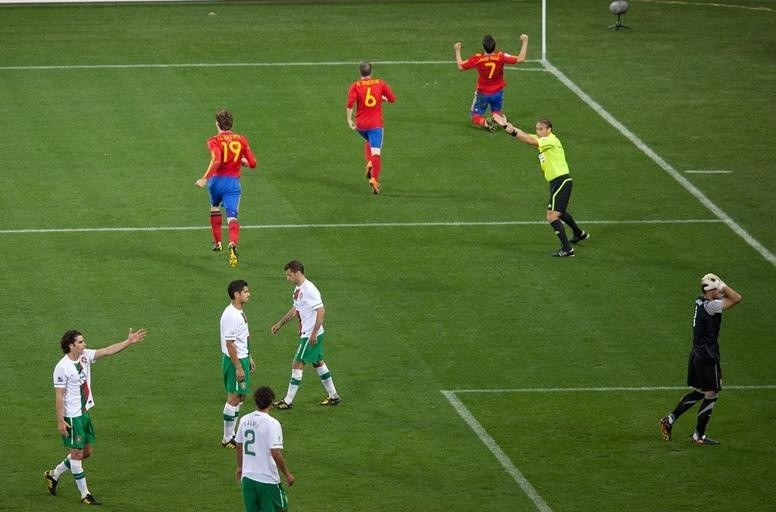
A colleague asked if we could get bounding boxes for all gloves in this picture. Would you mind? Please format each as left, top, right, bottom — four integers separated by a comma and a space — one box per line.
700, 273, 727, 291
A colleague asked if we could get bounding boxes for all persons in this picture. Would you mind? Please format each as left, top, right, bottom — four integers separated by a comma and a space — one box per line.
234, 386, 295, 512
346, 62, 397, 194
195, 108, 257, 268
660, 274, 742, 445
41, 327, 146, 506
271, 259, 342, 408
491, 113, 590, 257
454, 33, 529, 130
219, 280, 255, 446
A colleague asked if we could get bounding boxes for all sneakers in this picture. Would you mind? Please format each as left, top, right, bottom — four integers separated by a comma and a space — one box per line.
227, 244, 238, 267
79, 494, 102, 505
365, 161, 373, 178
271, 400, 291, 409
232, 436, 235, 439
320, 397, 340, 405
659, 416, 672, 441
550, 247, 575, 257
211, 242, 222, 251
692, 433, 720, 445
368, 177, 380, 194
220, 439, 236, 449
485, 119, 496, 132
42, 471, 57, 496
569, 230, 589, 244
